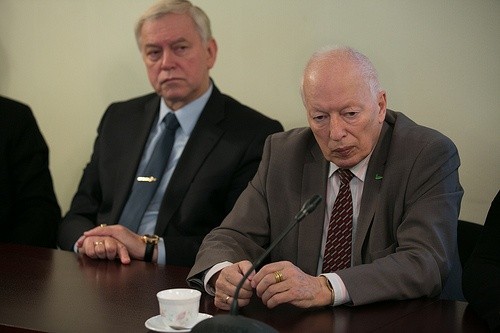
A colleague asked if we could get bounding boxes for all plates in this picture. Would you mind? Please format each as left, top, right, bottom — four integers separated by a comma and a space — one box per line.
145, 313, 214, 333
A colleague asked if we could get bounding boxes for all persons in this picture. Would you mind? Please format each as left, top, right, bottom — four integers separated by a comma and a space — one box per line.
0, 94, 62, 249
58, 0, 285, 265
187, 46, 465, 311
464, 193, 500, 333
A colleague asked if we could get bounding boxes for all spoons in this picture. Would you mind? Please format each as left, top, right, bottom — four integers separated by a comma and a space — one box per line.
170, 325, 193, 330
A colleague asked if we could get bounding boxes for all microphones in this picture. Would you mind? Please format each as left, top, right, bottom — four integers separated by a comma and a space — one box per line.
191, 193, 322, 333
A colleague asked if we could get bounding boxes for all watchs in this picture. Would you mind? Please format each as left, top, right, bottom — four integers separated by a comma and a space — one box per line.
139, 233, 159, 261
320, 275, 334, 303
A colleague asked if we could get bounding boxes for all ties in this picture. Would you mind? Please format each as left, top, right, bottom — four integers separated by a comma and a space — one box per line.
322, 169, 355, 273
117, 115, 179, 233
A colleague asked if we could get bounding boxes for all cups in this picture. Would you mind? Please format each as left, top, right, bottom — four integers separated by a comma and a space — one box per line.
156, 288, 201, 329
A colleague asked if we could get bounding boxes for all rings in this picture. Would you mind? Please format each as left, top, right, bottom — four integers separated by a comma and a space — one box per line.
272, 271, 283, 282
94, 241, 104, 246
225, 296, 230, 304
101, 224, 106, 227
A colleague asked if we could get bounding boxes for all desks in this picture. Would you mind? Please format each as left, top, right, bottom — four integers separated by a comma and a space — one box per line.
0, 242, 500, 333
